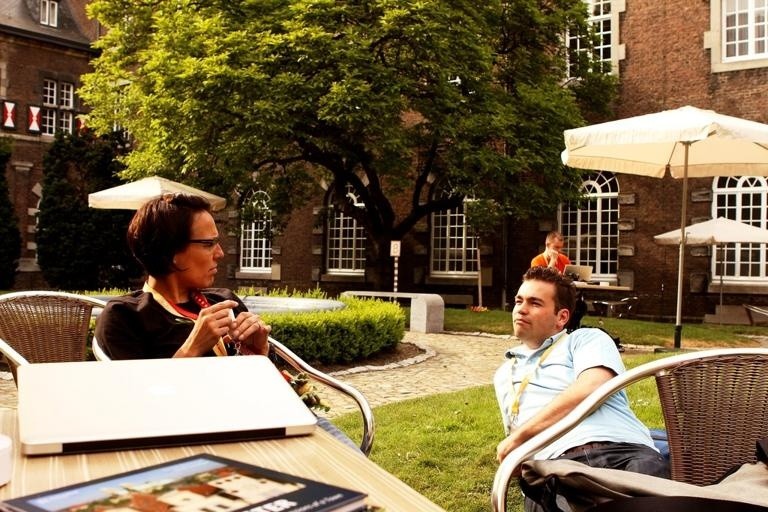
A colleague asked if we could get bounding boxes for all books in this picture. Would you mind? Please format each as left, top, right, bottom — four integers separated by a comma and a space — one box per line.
1, 451, 382, 512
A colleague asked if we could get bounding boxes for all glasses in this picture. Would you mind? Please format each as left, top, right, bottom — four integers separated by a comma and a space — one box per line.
190, 238, 221, 249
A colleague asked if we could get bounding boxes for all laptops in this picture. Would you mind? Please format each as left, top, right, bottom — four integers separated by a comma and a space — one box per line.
563, 264, 594, 282
16, 354, 318, 457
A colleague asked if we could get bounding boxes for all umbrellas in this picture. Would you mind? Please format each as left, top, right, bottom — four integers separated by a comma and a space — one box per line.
84, 175, 227, 213
652, 214, 768, 326
558, 105, 768, 347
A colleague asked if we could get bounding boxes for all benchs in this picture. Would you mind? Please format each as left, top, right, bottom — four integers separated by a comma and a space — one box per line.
339, 288, 445, 334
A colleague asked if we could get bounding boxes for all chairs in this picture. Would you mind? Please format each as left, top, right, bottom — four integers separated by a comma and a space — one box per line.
492, 343, 768, 510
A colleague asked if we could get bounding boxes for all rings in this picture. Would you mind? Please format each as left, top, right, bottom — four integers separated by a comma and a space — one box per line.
258, 322, 261, 330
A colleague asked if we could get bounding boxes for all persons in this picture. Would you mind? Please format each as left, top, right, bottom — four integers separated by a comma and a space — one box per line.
90, 191, 274, 363
488, 266, 675, 512
529, 229, 588, 330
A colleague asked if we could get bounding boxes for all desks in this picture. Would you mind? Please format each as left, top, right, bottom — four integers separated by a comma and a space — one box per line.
572, 281, 633, 352
2, 374, 451, 511
